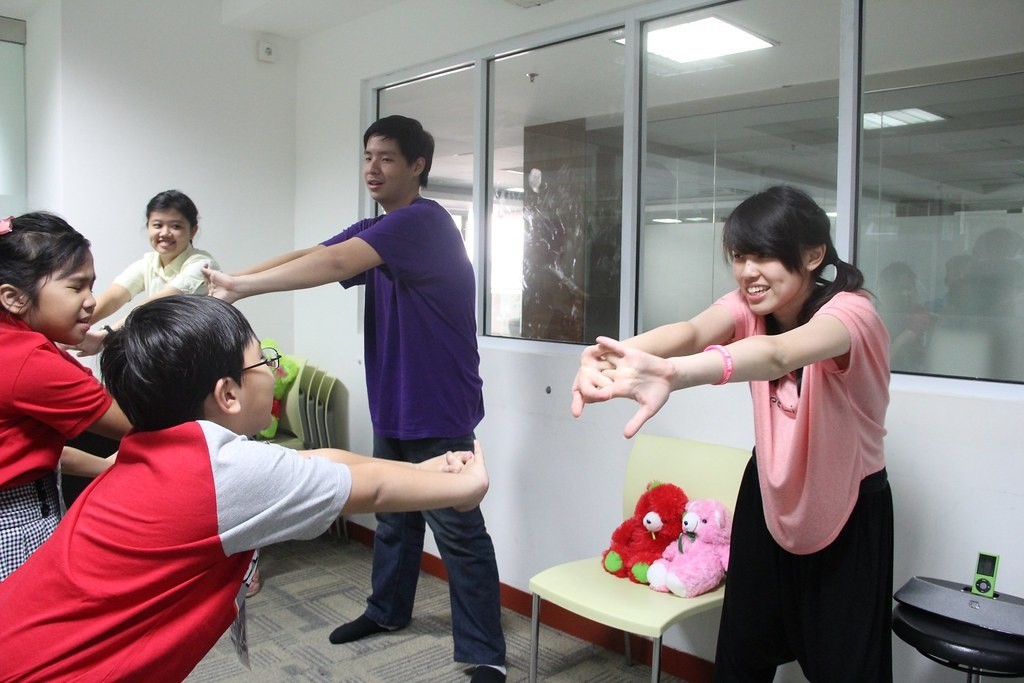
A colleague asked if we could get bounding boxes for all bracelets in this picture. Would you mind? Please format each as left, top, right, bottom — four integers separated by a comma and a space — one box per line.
705, 344, 734, 385
100, 324, 113, 333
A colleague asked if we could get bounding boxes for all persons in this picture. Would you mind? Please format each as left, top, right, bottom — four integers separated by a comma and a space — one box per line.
878, 225, 1024, 386
200, 115, 507, 683
0, 293, 490, 683
58, 189, 221, 357
570, 184, 893, 683
0, 211, 134, 581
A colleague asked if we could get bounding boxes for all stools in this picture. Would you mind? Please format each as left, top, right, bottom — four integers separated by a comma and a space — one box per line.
892, 603, 1024, 683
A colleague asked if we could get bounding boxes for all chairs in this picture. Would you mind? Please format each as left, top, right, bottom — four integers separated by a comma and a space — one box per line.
528, 433, 754, 683
257, 356, 337, 549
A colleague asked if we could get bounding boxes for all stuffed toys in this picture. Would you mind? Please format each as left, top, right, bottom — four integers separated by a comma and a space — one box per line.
257, 336, 299, 439
646, 499, 731, 598
601, 481, 688, 586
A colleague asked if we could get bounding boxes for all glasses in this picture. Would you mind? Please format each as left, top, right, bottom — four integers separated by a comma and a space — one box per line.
231, 346, 282, 378
